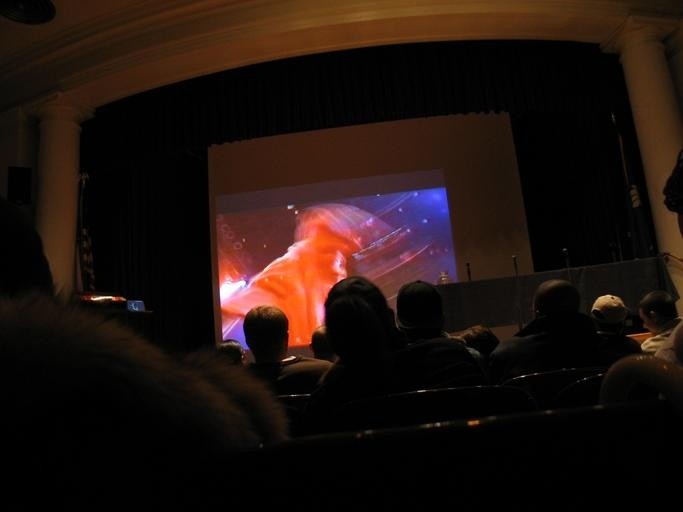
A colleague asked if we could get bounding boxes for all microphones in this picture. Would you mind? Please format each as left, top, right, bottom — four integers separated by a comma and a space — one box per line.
465, 262, 472, 281
562, 248, 569, 268
511, 255, 518, 275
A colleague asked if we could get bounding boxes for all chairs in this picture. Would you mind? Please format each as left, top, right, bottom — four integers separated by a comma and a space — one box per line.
1, 331, 683, 512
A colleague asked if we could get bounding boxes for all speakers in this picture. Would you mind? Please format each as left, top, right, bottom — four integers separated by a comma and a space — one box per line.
8, 166, 31, 204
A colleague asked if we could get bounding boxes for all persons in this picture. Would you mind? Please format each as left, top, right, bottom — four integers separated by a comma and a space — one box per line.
0, 217, 682, 512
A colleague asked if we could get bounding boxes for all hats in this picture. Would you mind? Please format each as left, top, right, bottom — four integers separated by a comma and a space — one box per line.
397, 280, 443, 325
591, 294, 627, 324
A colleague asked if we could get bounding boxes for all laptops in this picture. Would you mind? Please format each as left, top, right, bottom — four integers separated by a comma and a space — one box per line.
126, 299, 154, 315
